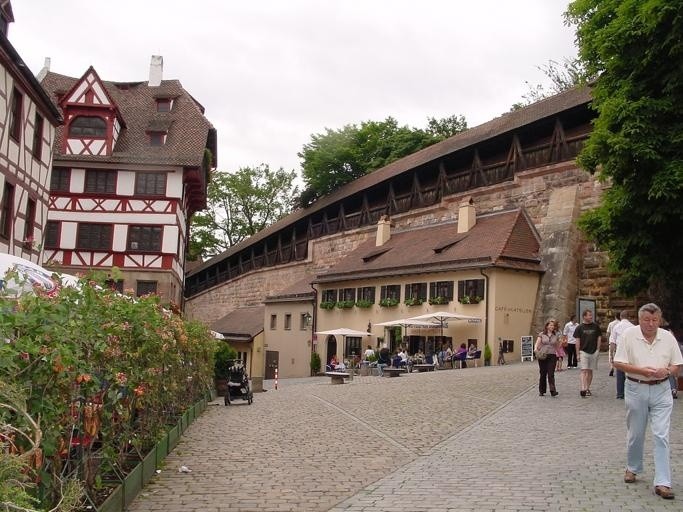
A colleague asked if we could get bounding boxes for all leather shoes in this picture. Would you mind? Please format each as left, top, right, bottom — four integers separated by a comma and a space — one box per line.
624, 469, 637, 483
655, 485, 676, 500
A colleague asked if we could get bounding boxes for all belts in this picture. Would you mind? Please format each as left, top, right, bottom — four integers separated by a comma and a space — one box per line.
626, 375, 670, 385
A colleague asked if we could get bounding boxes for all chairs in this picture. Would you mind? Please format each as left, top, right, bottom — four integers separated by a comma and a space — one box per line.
326, 345, 482, 373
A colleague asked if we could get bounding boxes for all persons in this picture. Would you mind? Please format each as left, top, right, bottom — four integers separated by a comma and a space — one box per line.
328, 342, 482, 373
609, 309, 635, 399
496, 341, 506, 366
550, 320, 565, 371
612, 303, 682, 499
533, 319, 560, 396
657, 317, 679, 399
573, 310, 602, 397
562, 314, 579, 369
605, 311, 621, 377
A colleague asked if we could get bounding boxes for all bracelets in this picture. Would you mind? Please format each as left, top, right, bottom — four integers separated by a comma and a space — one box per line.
665, 367, 671, 377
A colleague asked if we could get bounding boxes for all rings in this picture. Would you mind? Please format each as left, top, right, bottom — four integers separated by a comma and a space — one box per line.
647, 373, 649, 376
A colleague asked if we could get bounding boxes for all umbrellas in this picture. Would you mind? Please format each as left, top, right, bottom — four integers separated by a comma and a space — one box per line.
406, 310, 482, 351
371, 318, 443, 352
313, 326, 376, 365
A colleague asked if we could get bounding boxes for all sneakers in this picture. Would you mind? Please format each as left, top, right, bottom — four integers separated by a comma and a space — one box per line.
539, 392, 545, 396
580, 390, 592, 398
567, 366, 578, 369
616, 396, 625, 400
609, 370, 614, 376
671, 389, 678, 399
556, 368, 563, 371
551, 392, 559, 397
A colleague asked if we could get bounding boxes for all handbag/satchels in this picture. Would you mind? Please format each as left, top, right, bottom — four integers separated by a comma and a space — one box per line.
536, 351, 548, 360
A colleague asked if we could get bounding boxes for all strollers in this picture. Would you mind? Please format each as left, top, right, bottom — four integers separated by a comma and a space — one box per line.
222, 358, 255, 406
